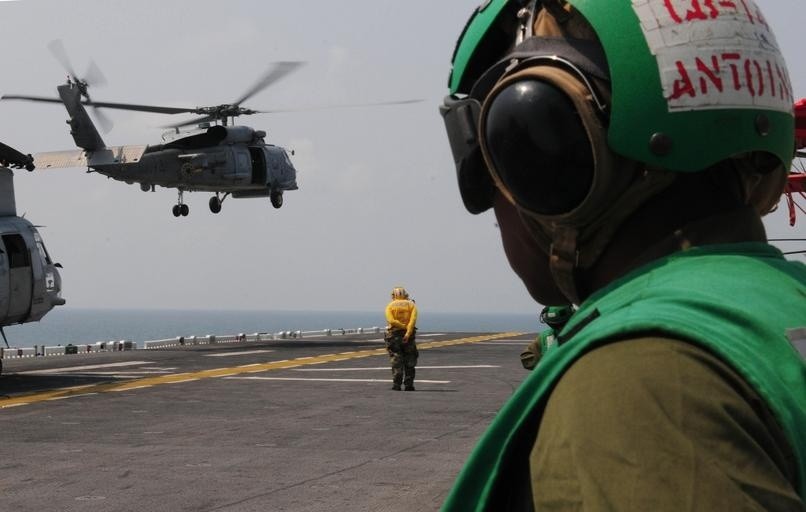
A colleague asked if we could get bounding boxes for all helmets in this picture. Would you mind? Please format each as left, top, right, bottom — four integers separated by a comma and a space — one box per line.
390, 287, 408, 300
438, 1, 796, 276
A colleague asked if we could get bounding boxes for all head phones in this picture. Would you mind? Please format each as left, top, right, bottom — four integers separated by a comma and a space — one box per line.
391, 287, 409, 298
473, 44, 617, 226
569, 305, 576, 314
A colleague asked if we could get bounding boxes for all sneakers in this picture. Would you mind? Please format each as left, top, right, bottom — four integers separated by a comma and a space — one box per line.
391, 386, 401, 391
404, 386, 415, 391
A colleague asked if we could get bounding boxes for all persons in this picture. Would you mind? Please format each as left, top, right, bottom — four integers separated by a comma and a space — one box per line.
384, 287, 419, 391
439, 0, 806, 512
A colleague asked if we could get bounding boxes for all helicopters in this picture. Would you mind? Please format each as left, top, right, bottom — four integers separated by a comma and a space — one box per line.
0, 142, 66, 348
0, 59, 424, 221
